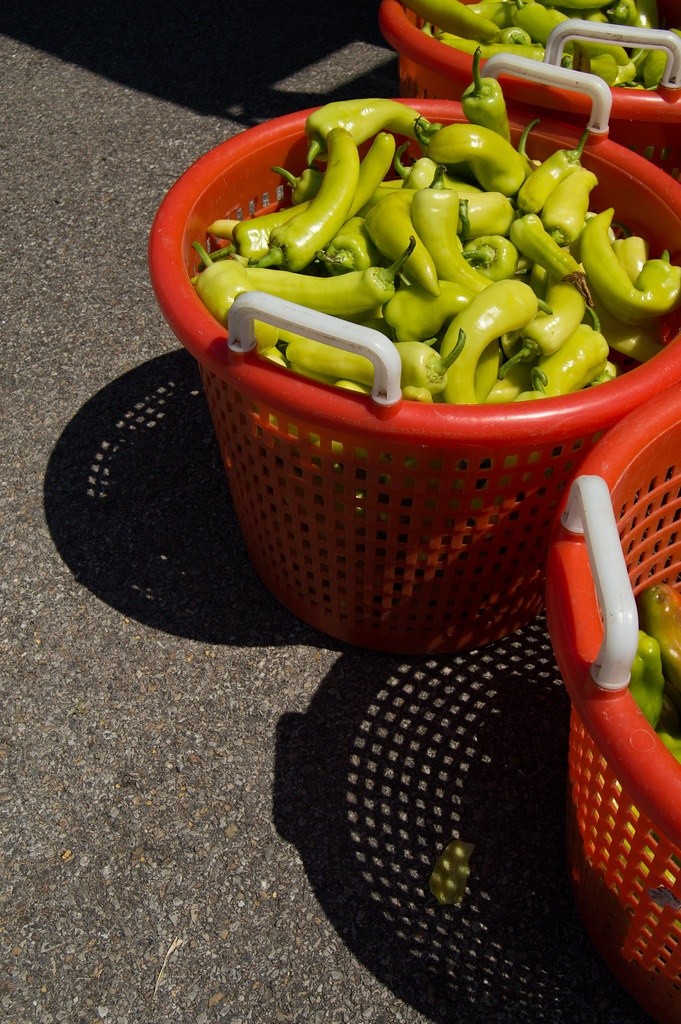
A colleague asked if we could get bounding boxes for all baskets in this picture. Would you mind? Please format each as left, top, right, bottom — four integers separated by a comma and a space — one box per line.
546, 379, 681, 1024
382, 0, 681, 180
147, 99, 681, 654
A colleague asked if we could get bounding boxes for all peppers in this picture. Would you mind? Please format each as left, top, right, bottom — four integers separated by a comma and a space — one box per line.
188, 44, 681, 642
397, 0, 681, 181
581, 579, 681, 994
